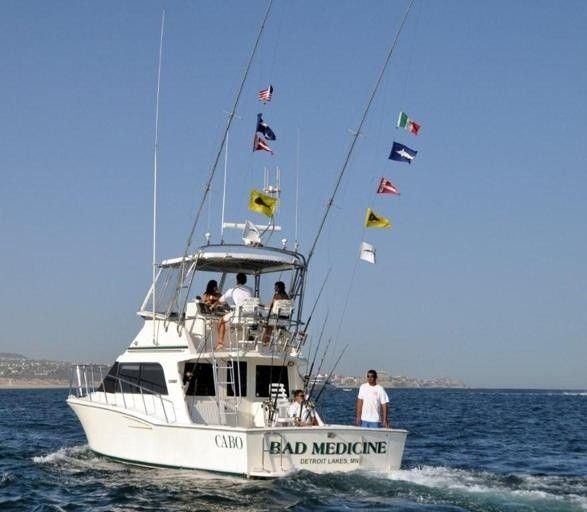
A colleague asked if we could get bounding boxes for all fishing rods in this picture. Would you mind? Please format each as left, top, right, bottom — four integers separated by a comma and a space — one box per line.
271, 266, 347, 428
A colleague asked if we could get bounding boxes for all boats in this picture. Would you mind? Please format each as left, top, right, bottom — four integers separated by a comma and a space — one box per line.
65, 3, 412, 478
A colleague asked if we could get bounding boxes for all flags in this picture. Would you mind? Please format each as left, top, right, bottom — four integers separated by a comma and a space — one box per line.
358, 109, 421, 265
242, 83, 280, 244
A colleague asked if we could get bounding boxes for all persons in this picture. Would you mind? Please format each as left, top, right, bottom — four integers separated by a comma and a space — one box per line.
357, 370, 391, 428
201, 271, 294, 352
286, 389, 319, 426
